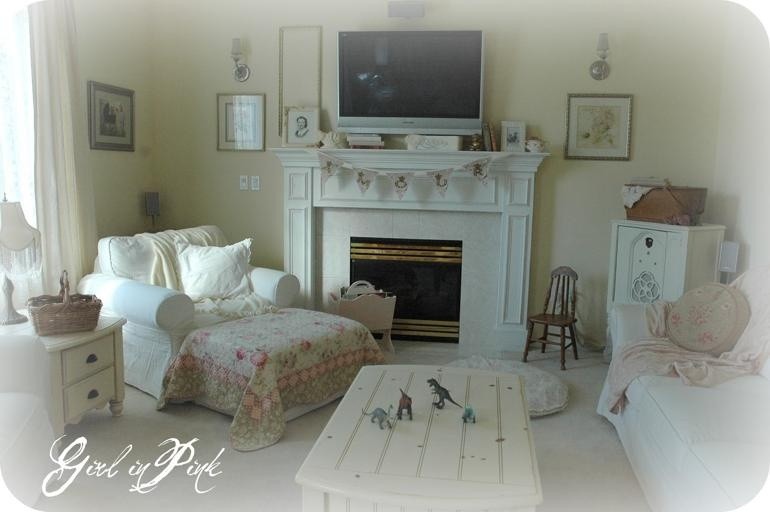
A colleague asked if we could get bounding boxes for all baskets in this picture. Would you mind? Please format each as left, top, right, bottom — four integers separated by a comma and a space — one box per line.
26, 269, 102, 334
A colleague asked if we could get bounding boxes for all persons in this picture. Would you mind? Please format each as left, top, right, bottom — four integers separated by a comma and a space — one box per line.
295, 116, 309, 137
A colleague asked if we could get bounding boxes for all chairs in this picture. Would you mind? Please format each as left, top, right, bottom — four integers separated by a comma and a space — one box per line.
76, 224, 301, 403
522, 266, 580, 370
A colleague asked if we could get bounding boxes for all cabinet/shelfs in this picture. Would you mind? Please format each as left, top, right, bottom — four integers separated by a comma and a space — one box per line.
0, 309, 128, 447
603, 220, 726, 364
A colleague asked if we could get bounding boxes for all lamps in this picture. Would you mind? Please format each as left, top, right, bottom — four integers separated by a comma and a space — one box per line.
231, 37, 250, 81
1, 192, 43, 326
589, 32, 610, 80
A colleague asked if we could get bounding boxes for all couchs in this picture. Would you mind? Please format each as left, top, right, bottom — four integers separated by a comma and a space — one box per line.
597, 264, 770, 512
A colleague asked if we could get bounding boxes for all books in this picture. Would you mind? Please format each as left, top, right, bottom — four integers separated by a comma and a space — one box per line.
347, 133, 384, 148
482, 122, 497, 152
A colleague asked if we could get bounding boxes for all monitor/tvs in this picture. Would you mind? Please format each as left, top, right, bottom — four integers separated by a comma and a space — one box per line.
337, 30, 486, 136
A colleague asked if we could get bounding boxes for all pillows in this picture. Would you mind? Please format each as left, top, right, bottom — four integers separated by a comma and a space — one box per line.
175, 237, 254, 300
669, 284, 750, 357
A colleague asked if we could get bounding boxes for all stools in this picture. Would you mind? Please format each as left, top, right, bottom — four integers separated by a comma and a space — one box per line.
155, 307, 384, 453
0, 334, 60, 512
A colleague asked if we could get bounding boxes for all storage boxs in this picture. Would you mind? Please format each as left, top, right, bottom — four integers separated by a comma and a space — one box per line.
326, 291, 397, 353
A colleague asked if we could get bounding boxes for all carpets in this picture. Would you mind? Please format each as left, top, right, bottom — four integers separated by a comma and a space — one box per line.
444, 354, 569, 419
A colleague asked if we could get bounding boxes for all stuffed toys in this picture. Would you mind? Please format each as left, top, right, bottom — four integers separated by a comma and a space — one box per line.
427, 378, 463, 409
361, 404, 394, 429
397, 388, 413, 420
462, 407, 476, 423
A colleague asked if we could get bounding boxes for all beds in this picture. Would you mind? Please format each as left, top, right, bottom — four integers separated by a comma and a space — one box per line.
295, 364, 542, 512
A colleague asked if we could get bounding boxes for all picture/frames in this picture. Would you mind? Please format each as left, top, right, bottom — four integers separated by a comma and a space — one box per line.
500, 120, 525, 153
88, 80, 134, 153
216, 93, 265, 152
564, 93, 633, 161
283, 105, 320, 147
